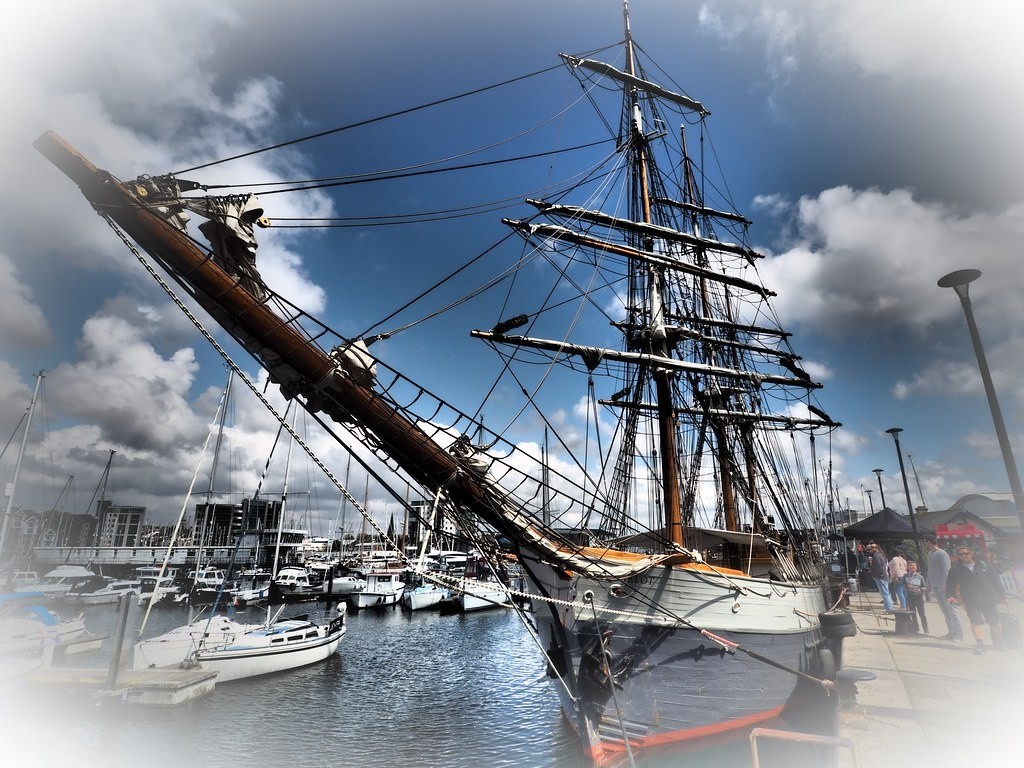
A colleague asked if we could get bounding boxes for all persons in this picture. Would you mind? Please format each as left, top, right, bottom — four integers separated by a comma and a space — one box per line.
838, 541, 908, 615
945, 546, 1010, 656
923, 534, 965, 643
902, 561, 929, 634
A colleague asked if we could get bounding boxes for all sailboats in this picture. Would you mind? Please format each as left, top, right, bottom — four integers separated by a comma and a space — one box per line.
28, 0, 859, 767
0, 363, 517, 684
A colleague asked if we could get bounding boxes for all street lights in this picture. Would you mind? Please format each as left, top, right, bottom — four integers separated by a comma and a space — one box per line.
864, 489, 874, 515
937, 266, 1024, 534
883, 427, 931, 604
871, 468, 886, 509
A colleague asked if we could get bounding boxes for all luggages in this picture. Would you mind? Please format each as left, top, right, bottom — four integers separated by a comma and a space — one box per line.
996, 603, 1023, 649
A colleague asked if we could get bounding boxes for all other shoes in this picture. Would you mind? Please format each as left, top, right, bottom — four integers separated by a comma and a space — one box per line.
941, 633, 952, 640
880, 600, 884, 603
994, 644, 1008, 652
952, 632, 961, 642
916, 630, 921, 634
975, 646, 985, 654
880, 612, 894, 615
924, 632, 931, 637
895, 607, 906, 611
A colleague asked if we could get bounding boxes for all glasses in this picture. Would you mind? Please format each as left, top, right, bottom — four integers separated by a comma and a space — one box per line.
871, 547, 874, 548
959, 553, 969, 556
